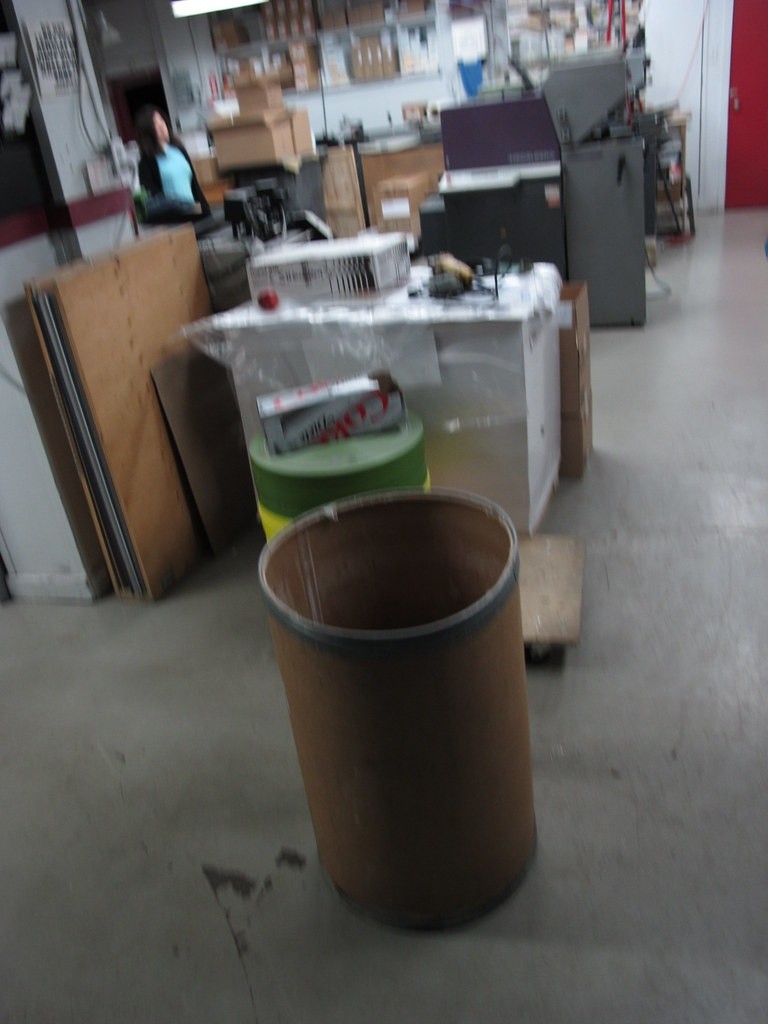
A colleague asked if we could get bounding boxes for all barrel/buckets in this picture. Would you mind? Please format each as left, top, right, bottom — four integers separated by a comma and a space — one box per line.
249, 408, 430, 546
258, 487, 539, 932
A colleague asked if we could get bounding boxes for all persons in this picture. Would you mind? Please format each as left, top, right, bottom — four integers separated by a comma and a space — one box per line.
136, 110, 212, 222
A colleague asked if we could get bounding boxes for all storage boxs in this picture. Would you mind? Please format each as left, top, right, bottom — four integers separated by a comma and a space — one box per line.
254, 367, 409, 457
551, 277, 592, 480
206, 1, 437, 170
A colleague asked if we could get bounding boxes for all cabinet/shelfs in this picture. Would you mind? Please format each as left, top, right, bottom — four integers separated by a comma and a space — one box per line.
213, 1, 451, 103
217, 261, 561, 532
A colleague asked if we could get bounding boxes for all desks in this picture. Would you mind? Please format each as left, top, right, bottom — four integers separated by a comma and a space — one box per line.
363, 138, 445, 237
